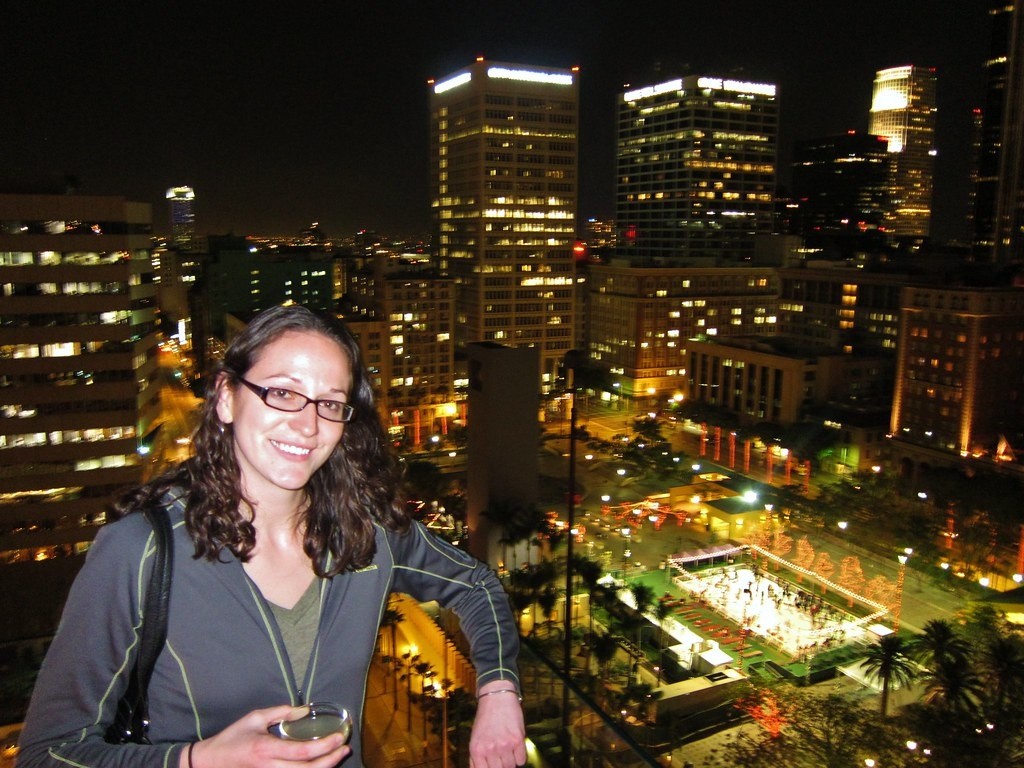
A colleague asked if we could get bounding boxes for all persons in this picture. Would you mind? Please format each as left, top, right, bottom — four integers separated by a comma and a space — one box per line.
12, 299, 528, 768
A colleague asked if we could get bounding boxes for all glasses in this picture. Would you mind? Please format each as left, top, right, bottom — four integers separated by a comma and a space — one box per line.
221, 369, 359, 423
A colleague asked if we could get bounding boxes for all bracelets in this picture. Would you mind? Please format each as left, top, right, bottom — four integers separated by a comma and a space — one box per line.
187, 735, 200, 768
477, 689, 522, 701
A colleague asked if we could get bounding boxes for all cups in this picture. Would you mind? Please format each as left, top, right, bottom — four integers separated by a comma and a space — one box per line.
279, 701, 352, 745
